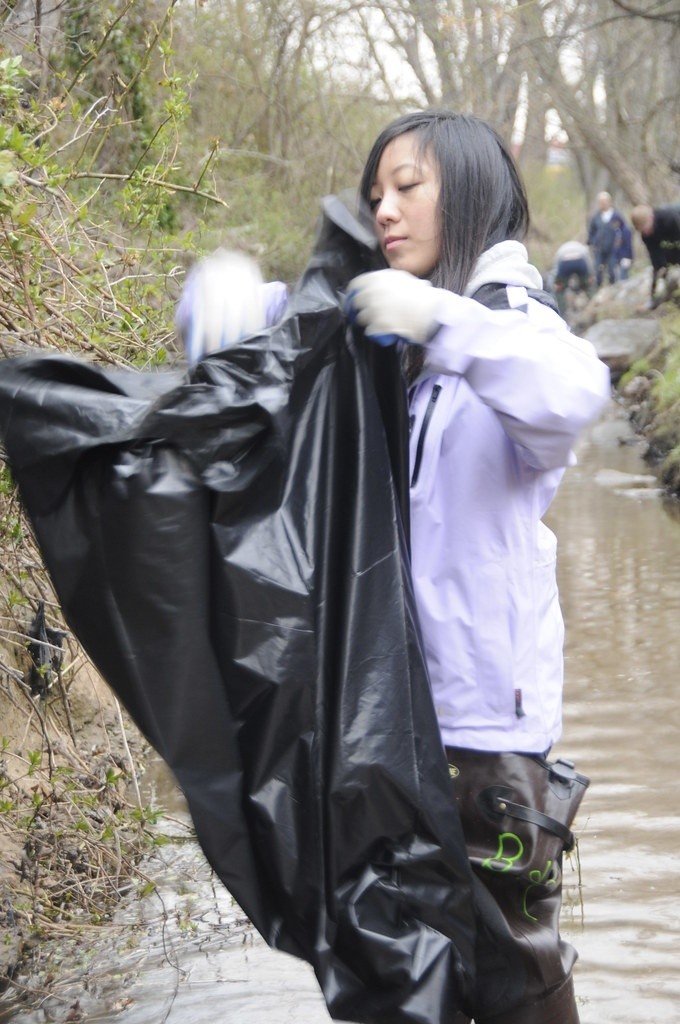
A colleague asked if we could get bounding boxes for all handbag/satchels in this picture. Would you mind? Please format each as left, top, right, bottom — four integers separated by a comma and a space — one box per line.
3, 199, 529, 1024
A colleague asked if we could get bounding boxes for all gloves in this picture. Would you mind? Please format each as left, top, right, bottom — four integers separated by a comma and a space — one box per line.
348, 267, 433, 347
195, 253, 268, 367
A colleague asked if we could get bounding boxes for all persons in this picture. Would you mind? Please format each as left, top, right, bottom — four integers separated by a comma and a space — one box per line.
546, 239, 597, 316
172, 113, 610, 1024
606, 214, 634, 280
586, 190, 621, 289
631, 201, 680, 311
